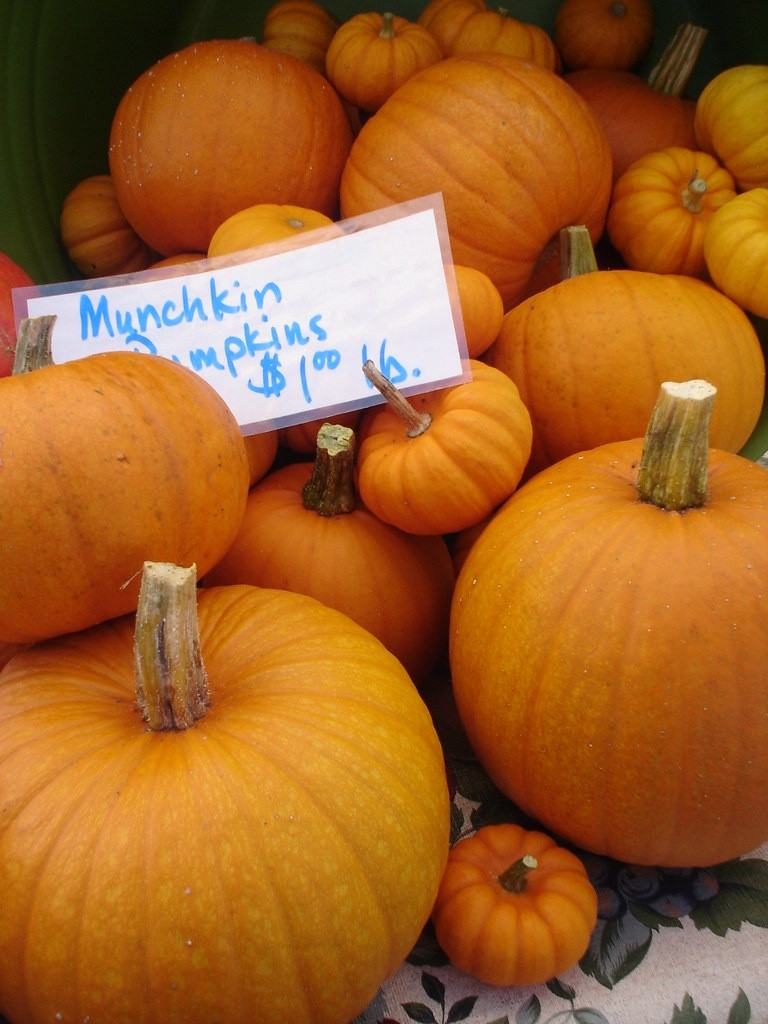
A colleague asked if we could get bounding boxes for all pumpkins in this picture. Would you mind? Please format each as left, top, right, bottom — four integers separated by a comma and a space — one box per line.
0, 0, 768, 1024
